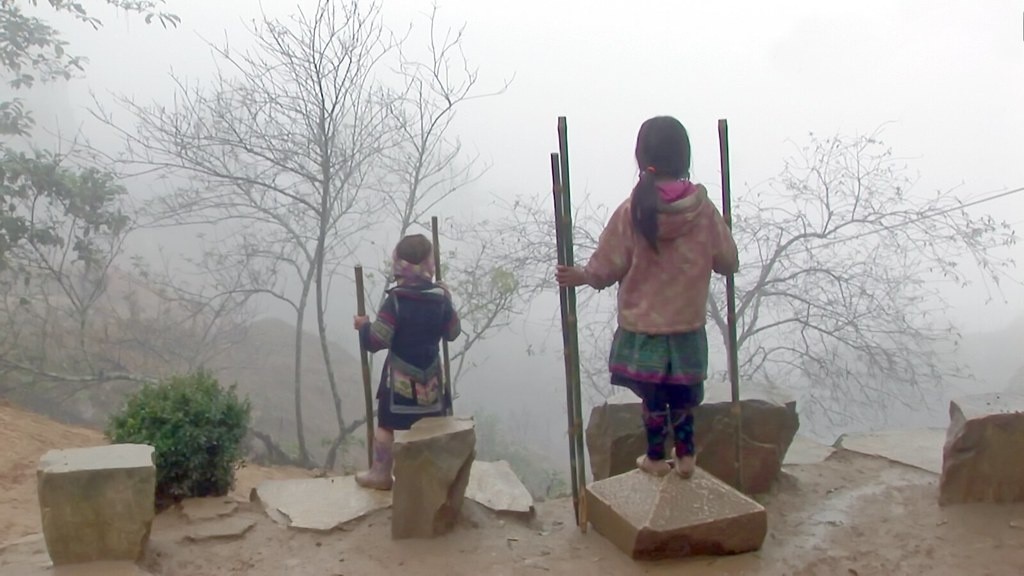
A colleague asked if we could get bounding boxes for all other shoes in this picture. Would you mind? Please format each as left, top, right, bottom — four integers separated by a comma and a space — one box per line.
671, 446, 696, 478
636, 454, 672, 476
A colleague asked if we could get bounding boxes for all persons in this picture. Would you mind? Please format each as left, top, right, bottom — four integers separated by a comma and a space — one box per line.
354, 233, 461, 491
555, 116, 740, 479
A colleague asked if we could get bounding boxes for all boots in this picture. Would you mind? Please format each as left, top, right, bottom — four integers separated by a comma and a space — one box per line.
355, 439, 394, 490
